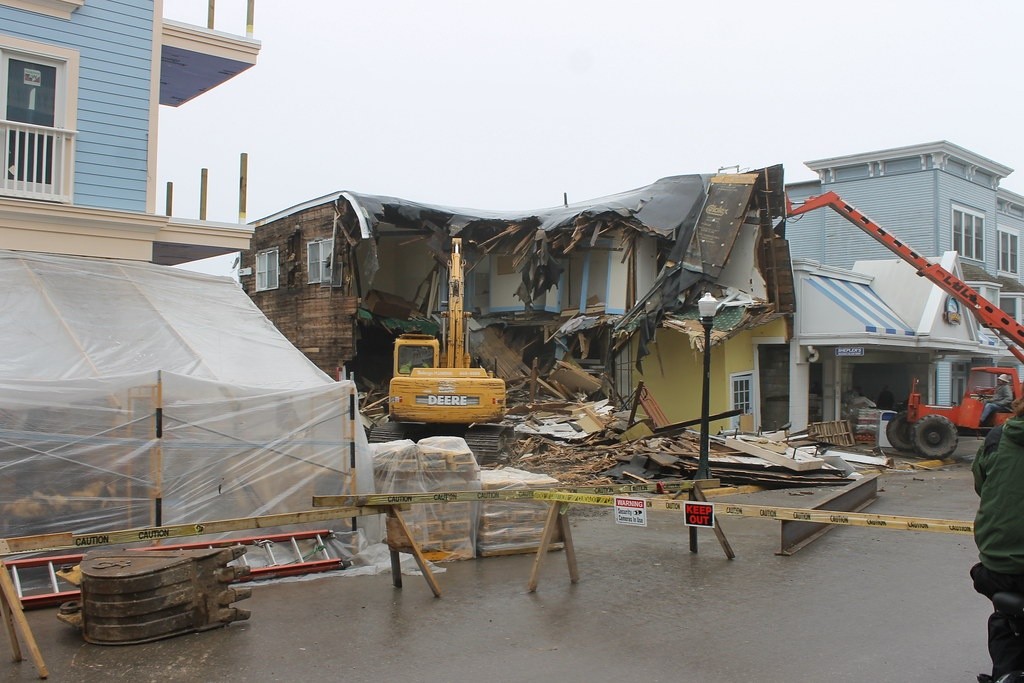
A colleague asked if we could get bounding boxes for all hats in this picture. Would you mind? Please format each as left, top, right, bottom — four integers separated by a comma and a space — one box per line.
997, 374, 1010, 382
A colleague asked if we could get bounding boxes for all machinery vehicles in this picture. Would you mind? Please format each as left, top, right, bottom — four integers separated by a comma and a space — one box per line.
389, 237, 507, 424
786, 191, 1024, 460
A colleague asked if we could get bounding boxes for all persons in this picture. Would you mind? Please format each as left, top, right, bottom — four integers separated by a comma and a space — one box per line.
970, 396, 1024, 587
978, 372, 1014, 424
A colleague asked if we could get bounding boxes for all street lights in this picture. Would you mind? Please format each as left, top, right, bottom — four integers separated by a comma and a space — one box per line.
696, 293, 722, 479
